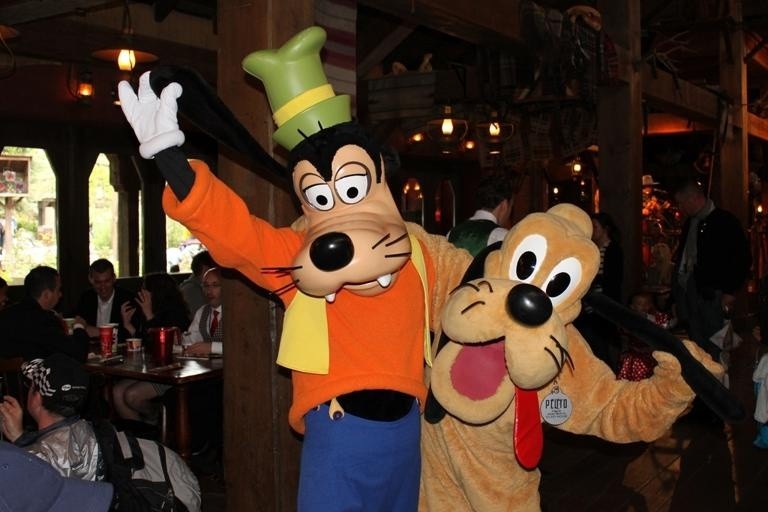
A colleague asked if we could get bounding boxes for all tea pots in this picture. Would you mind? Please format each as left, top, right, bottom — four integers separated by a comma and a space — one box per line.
147, 328, 182, 361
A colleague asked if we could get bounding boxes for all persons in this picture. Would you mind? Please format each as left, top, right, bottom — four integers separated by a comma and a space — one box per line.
443, 170, 507, 255
590, 171, 768, 381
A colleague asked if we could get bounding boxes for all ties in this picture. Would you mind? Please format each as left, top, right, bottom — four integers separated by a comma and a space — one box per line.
210, 310, 220, 338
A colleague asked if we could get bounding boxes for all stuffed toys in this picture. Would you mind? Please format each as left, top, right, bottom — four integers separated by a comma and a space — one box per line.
292, 203, 748, 512
112, 22, 473, 511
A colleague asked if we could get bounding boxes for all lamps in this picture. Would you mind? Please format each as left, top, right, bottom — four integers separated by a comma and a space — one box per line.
420, 95, 516, 159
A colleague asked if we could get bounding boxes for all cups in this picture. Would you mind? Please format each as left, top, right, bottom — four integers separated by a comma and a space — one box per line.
99, 326, 113, 355
105, 323, 119, 353
126, 338, 142, 353
63, 318, 75, 334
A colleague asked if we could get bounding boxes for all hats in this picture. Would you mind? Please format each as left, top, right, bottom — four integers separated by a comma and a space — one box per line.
21, 356, 88, 407
